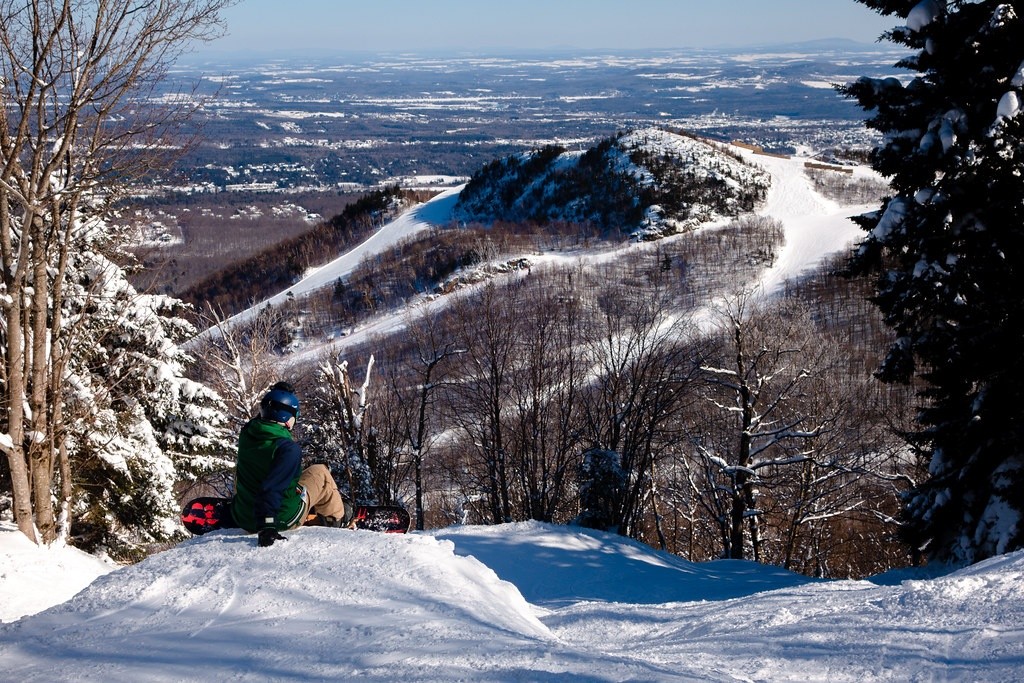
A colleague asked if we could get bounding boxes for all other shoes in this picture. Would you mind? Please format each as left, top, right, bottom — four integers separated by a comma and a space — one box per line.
318, 498, 355, 527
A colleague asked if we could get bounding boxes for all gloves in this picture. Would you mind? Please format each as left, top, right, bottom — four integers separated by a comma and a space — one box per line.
258, 522, 286, 548
269, 382, 295, 394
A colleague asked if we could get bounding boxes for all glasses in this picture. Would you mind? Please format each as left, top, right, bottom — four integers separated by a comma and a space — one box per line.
295, 410, 299, 425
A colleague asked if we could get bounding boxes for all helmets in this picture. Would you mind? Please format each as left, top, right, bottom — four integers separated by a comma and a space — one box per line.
259, 390, 299, 422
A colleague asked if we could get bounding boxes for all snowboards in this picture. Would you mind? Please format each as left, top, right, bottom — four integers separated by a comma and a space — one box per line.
182, 497, 411, 534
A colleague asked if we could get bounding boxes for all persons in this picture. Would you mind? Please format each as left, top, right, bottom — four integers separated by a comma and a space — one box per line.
231, 381, 353, 547
